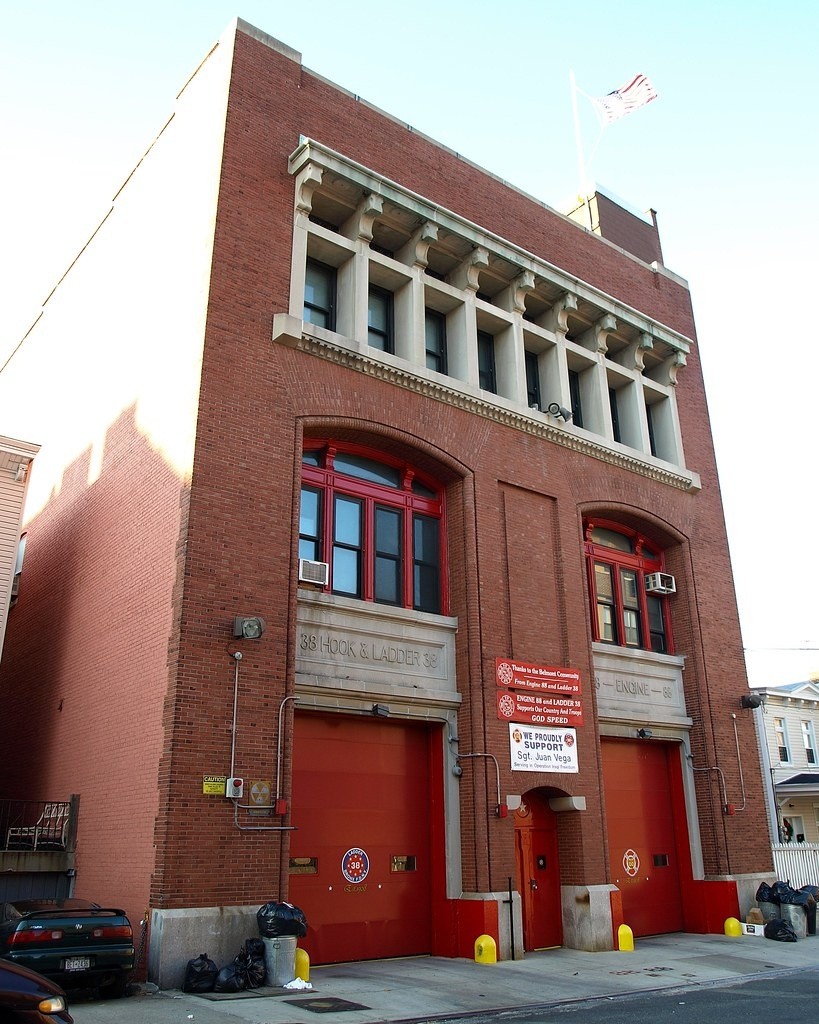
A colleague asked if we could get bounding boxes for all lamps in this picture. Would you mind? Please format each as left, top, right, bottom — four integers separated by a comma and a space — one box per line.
638, 728, 653, 739
555, 407, 575, 422
531, 402, 540, 411
233, 615, 267, 639
742, 694, 765, 709
543, 403, 559, 414
372, 704, 389, 718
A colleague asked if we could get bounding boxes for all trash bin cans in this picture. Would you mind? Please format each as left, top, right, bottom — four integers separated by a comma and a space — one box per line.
758, 900, 780, 923
780, 904, 807, 938
262, 936, 297, 985
808, 903, 819, 934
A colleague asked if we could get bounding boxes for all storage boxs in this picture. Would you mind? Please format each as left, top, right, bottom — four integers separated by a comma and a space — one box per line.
742, 906, 768, 937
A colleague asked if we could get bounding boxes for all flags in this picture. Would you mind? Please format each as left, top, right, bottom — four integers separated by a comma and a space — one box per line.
603, 71, 656, 122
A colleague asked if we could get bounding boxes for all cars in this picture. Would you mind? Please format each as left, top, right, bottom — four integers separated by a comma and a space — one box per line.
0, 956, 74, 1024
0, 896, 135, 1004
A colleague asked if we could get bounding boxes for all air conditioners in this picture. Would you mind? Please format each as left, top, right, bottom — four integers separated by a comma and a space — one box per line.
644, 572, 677, 595
299, 558, 329, 585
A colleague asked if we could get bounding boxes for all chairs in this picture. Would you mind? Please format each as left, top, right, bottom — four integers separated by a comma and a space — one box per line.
6, 803, 70, 851
